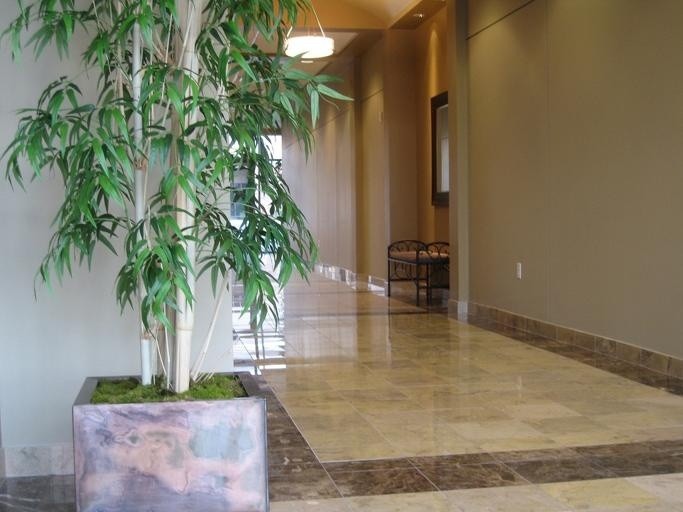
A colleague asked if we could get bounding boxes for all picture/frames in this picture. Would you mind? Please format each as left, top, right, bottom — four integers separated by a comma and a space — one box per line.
427, 89, 455, 209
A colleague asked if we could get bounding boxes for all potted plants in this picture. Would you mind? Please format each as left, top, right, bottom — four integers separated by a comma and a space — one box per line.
2, 0, 360, 511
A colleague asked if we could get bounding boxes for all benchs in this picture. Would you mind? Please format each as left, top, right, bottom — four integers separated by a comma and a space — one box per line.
386, 235, 451, 309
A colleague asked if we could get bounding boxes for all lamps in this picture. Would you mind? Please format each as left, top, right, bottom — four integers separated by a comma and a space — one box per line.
279, 1, 335, 65
